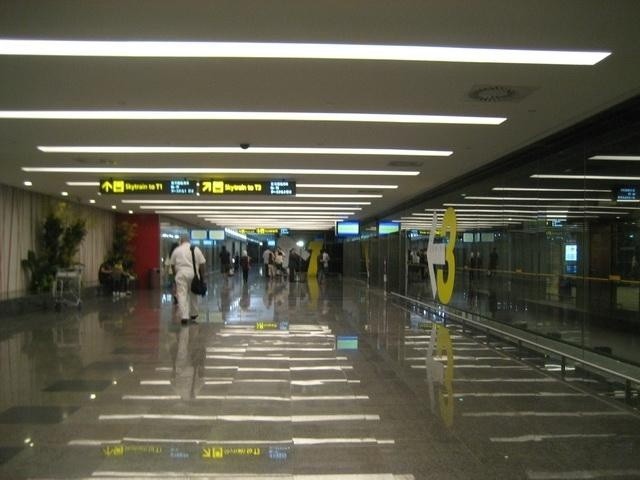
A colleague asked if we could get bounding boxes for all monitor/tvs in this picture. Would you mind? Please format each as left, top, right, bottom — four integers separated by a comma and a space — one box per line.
335, 336, 360, 352
379, 222, 401, 235
564, 243, 578, 263
335, 220, 360, 237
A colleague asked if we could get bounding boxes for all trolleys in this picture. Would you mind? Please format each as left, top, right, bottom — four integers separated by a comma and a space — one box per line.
51, 320, 84, 369
50, 263, 86, 312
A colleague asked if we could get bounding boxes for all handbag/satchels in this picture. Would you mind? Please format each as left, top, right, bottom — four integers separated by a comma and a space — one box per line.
191, 275, 207, 294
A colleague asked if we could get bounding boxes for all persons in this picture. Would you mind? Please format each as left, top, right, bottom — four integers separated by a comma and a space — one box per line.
98, 255, 136, 296
408, 246, 502, 291
170, 232, 206, 323
218, 245, 230, 282
260, 244, 331, 283
239, 250, 251, 283
218, 283, 332, 321
170, 325, 206, 410
161, 241, 180, 303
